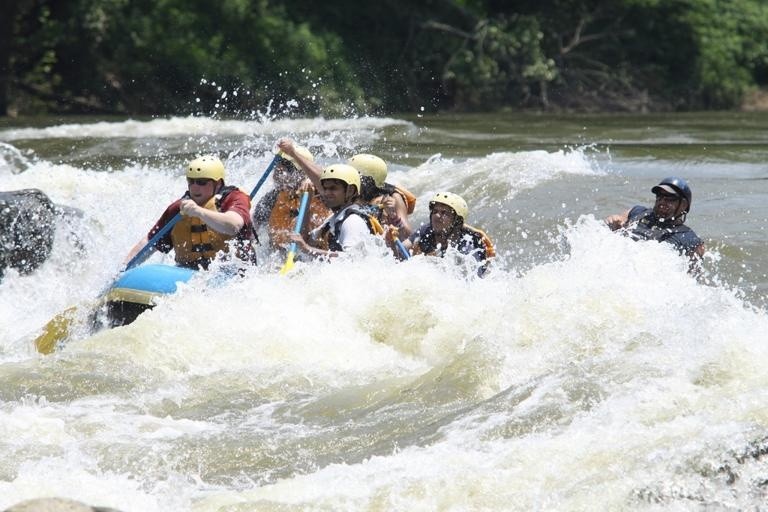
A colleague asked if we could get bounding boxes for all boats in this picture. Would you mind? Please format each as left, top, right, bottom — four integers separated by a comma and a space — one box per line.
104, 261, 199, 327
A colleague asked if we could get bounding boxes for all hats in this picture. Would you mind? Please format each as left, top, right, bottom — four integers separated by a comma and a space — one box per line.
650, 184, 680, 198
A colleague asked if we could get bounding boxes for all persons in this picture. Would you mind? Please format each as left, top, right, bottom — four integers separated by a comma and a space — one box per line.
339, 150, 418, 261
594, 174, 708, 274
267, 159, 394, 275
249, 136, 336, 267
383, 189, 497, 283
106, 155, 260, 290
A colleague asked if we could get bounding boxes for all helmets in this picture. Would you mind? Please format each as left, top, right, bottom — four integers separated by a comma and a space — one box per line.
429, 191, 468, 220
658, 176, 691, 213
319, 163, 360, 198
348, 153, 387, 188
272, 141, 313, 172
186, 155, 225, 186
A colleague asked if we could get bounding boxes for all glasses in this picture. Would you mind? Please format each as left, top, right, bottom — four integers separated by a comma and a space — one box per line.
655, 193, 679, 202
188, 178, 208, 185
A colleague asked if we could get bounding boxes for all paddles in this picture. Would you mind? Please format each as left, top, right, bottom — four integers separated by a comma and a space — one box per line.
35, 209, 185, 356
